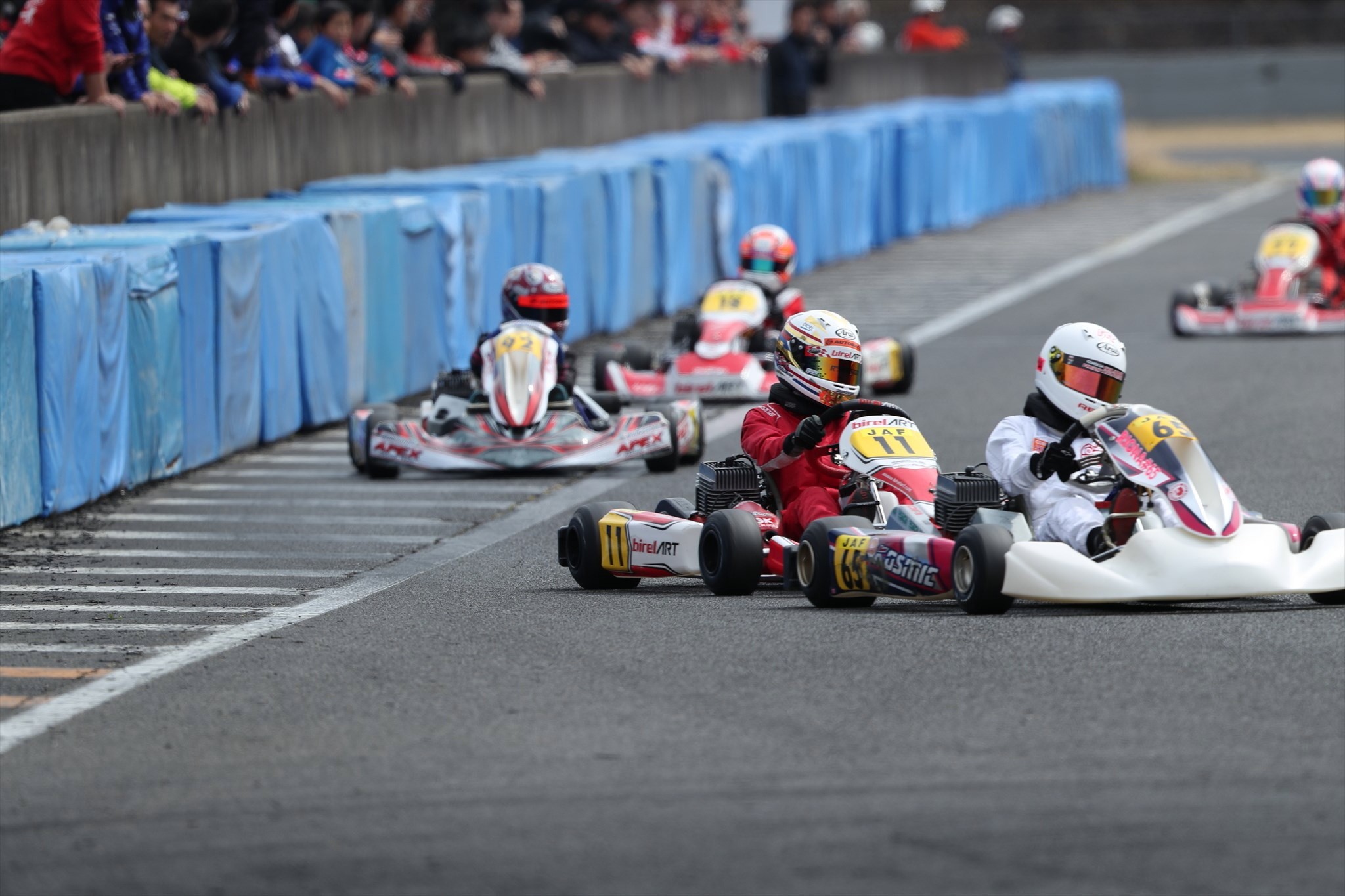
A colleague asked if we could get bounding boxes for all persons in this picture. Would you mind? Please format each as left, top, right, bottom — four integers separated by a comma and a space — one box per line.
658, 225, 805, 375
1208, 157, 1345, 311
740, 309, 864, 540
0, 0, 969, 125
427, 261, 579, 433
986, 323, 1330, 562
987, 6, 1026, 83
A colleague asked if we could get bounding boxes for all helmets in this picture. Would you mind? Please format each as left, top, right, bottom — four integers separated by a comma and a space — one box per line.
501, 262, 570, 337
736, 223, 798, 295
1034, 322, 1127, 428
1294, 158, 1345, 225
773, 309, 863, 406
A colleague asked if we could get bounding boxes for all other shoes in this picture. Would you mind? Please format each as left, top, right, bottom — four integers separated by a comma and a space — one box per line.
1094, 488, 1140, 557
844, 488, 876, 523
550, 384, 569, 411
469, 388, 489, 412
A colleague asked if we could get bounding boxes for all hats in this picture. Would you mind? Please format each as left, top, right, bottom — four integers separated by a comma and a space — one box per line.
991, 5, 1022, 33
908, 0, 948, 15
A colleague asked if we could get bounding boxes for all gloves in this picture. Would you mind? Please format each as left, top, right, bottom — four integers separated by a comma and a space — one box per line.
783, 414, 826, 457
1029, 442, 1076, 481
